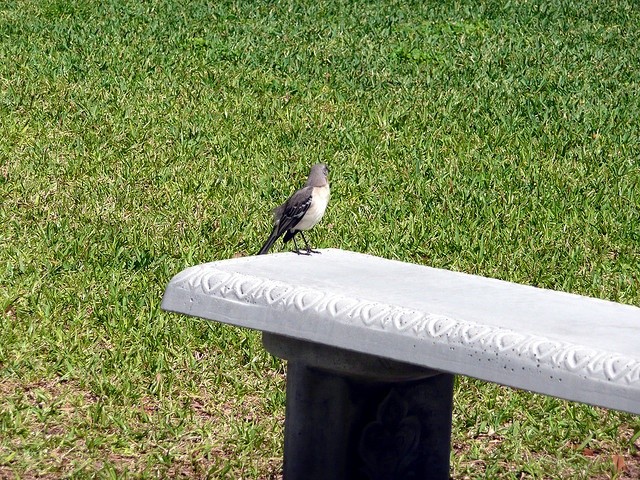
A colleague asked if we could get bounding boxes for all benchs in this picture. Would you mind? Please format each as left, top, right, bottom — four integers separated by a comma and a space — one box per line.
161, 247, 640, 479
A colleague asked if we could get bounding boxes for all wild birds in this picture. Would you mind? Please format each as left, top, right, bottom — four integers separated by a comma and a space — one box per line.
257, 162, 332, 256
